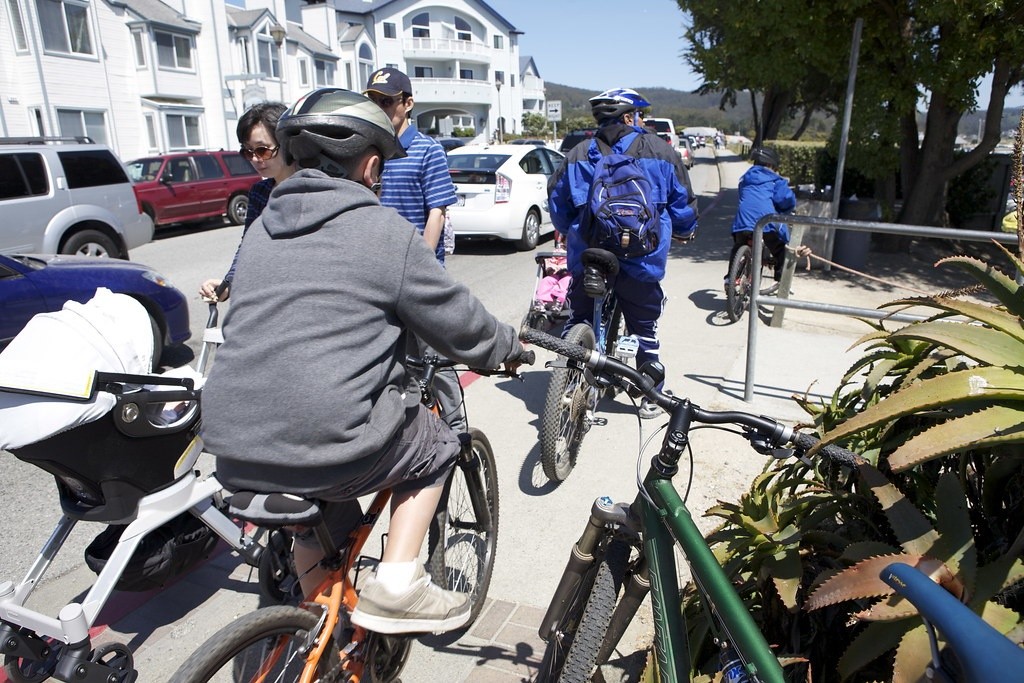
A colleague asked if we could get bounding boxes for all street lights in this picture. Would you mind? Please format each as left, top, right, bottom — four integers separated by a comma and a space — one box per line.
495, 80, 503, 145
270, 22, 285, 105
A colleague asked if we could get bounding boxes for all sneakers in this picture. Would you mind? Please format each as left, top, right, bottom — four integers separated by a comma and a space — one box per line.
639, 390, 674, 419
550, 300, 563, 315
530, 299, 549, 313
350, 558, 472, 635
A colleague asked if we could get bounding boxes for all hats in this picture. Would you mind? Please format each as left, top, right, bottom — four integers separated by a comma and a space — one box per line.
360, 67, 413, 98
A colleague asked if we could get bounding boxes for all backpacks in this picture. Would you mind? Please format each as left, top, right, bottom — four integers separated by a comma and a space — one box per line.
581, 130, 661, 257
443, 206, 455, 255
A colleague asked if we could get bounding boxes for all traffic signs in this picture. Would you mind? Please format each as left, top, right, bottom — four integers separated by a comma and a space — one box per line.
547, 101, 561, 121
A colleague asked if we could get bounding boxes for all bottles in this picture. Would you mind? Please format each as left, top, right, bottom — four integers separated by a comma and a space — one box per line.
716, 649, 751, 683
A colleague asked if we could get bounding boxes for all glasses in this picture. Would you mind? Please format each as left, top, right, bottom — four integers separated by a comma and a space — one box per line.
636, 109, 644, 118
239, 144, 280, 160
368, 95, 405, 108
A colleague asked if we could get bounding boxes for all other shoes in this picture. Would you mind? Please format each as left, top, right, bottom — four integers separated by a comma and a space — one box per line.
774, 282, 795, 295
724, 277, 737, 296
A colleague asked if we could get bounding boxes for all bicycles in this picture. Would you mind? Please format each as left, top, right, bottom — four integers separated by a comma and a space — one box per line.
517, 324, 1023, 683
727, 237, 780, 323
165, 350, 536, 683
540, 236, 697, 483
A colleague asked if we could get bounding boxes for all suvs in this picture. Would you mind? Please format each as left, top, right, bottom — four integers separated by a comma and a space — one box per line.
124, 148, 264, 226
0, 136, 155, 260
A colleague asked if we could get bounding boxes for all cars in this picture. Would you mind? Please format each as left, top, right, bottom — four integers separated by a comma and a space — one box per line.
438, 128, 600, 168
0, 252, 192, 374
444, 145, 567, 251
642, 118, 706, 170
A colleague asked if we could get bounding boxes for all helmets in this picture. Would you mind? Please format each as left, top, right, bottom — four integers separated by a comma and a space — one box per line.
752, 147, 780, 170
275, 89, 409, 162
588, 88, 651, 122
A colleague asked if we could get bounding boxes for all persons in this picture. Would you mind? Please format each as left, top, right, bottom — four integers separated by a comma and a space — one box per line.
724, 147, 796, 294
199, 102, 301, 303
547, 87, 698, 418
360, 68, 467, 432
202, 87, 527, 664
695, 132, 721, 149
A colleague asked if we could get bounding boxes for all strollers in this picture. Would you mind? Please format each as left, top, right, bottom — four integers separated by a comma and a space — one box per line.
0, 278, 301, 683
521, 229, 573, 333
